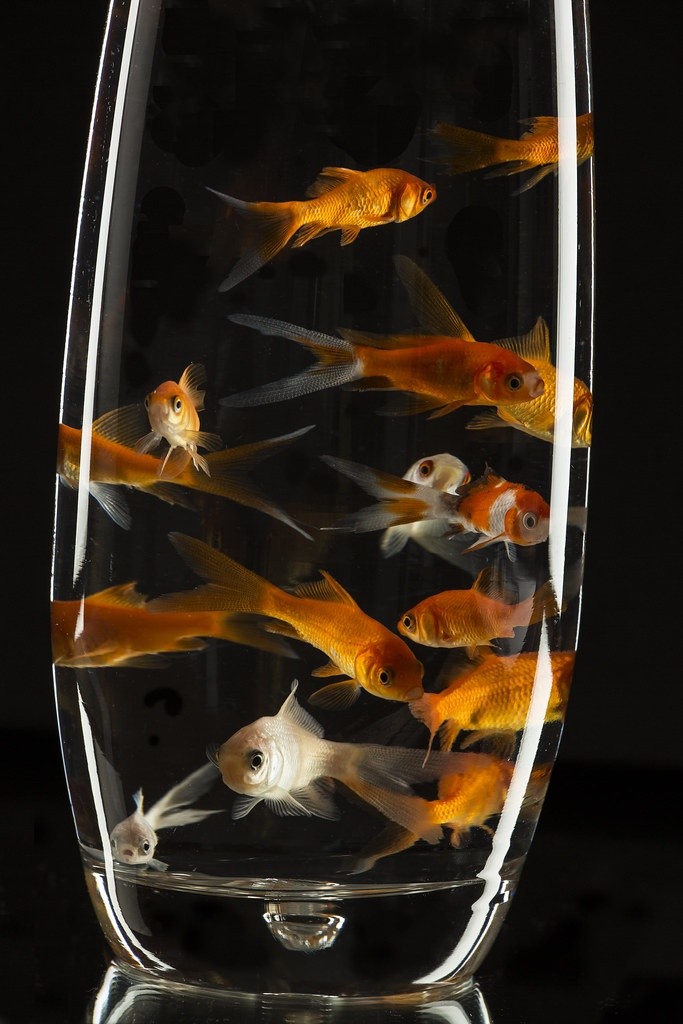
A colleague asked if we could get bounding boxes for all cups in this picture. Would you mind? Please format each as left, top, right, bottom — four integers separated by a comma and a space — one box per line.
55, 0, 594, 1004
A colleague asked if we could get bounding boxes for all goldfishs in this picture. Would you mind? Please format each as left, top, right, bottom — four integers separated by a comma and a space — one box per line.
51, 533, 576, 870
216, 254, 592, 449
437, 114, 594, 195
205, 166, 437, 293
57, 363, 314, 539
320, 453, 551, 579
